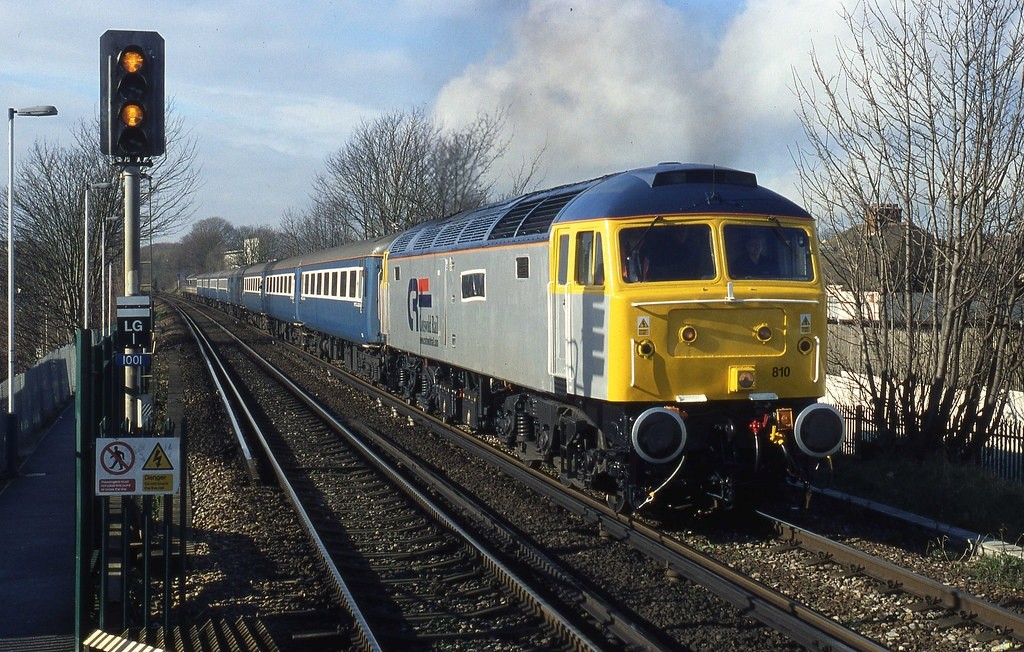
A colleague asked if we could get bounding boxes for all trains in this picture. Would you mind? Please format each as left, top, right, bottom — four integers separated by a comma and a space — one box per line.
140, 262, 153, 295
181, 163, 846, 526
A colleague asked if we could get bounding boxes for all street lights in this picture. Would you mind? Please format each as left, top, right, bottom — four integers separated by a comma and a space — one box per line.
81, 181, 115, 331
8, 104, 58, 479
100, 215, 119, 336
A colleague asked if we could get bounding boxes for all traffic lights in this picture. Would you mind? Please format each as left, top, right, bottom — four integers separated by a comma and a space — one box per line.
99, 30, 166, 160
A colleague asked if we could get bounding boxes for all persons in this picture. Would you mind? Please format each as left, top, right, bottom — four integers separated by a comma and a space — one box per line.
733, 230, 773, 278
